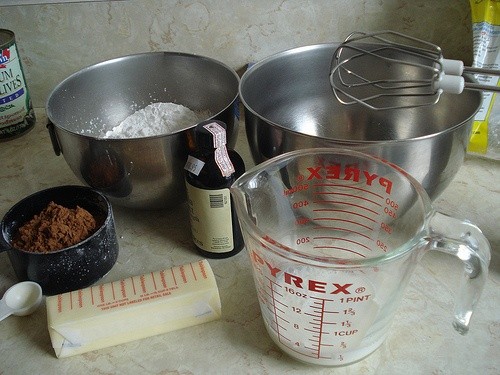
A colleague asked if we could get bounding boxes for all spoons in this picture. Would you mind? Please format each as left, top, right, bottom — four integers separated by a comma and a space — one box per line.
0, 281, 43, 323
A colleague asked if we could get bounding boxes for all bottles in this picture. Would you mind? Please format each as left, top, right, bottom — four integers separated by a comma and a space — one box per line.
0, 28, 36, 142
184, 125, 250, 259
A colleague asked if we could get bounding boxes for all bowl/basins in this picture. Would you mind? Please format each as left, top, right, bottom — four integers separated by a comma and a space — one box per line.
240, 40, 485, 231
0, 185, 119, 295
45, 51, 241, 209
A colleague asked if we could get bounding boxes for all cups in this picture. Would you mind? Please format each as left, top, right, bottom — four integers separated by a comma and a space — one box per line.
227, 147, 492, 367
